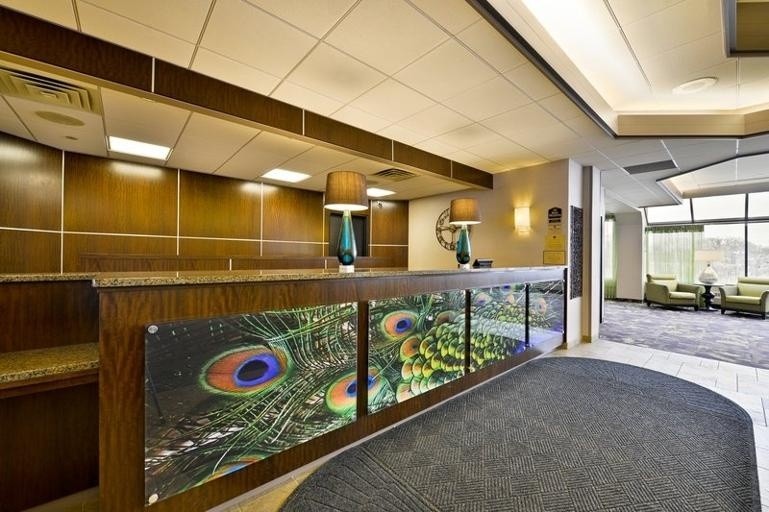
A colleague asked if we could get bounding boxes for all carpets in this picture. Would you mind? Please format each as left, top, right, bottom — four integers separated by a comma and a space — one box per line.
599, 299, 767, 370
270, 355, 765, 509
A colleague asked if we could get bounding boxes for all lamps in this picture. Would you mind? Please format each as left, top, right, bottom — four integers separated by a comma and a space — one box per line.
447, 197, 484, 264
323, 171, 369, 264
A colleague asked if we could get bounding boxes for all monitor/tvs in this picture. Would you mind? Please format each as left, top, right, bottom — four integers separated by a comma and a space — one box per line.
472, 258, 493, 266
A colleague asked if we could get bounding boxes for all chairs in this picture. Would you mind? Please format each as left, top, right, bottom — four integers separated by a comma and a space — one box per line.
643, 273, 769, 321
